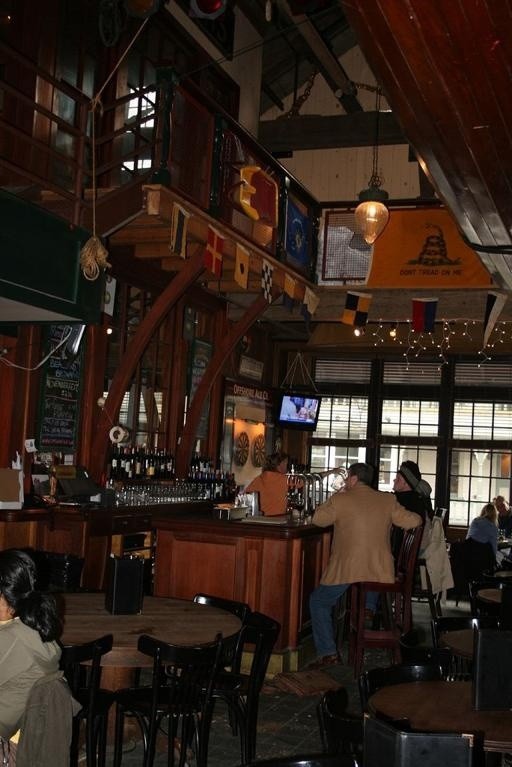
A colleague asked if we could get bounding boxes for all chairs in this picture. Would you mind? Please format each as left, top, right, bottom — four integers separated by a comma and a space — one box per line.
455, 540, 493, 607
316, 687, 363, 751
398, 628, 429, 666
192, 594, 251, 621
347, 525, 424, 681
431, 615, 492, 649
56, 634, 115, 766
114, 634, 222, 767
413, 508, 447, 618
179, 612, 281, 766
359, 657, 443, 711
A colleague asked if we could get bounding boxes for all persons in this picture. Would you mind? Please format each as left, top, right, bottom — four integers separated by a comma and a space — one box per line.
1, 545, 63, 766
310, 462, 422, 667
394, 460, 426, 526
466, 503, 499, 556
298, 406, 310, 419
415, 477, 455, 597
243, 451, 346, 518
494, 496, 512, 538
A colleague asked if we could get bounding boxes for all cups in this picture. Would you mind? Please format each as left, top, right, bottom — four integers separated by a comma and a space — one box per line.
498, 529, 506, 541
440, 654, 459, 700
295, 498, 304, 521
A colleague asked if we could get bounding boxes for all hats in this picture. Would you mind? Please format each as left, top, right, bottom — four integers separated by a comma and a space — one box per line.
400, 460, 421, 490
415, 478, 432, 496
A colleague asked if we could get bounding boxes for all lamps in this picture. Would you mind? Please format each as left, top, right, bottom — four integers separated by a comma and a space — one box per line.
355, 189, 389, 246
389, 326, 398, 338
354, 327, 364, 338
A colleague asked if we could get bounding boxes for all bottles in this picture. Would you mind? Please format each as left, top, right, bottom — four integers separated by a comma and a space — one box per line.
110, 446, 119, 477
83, 470, 101, 489
118, 446, 125, 477
160, 450, 164, 477
109, 475, 113, 488
188, 452, 224, 480
153, 453, 156, 466
144, 449, 149, 473
232, 473, 237, 498
128, 470, 132, 478
167, 448, 172, 478
163, 448, 167, 477
49, 466, 58, 496
157, 452, 160, 474
131, 447, 136, 467
172, 449, 175, 479
149, 449, 154, 475
128, 444, 132, 449
135, 444, 142, 474
126, 450, 131, 473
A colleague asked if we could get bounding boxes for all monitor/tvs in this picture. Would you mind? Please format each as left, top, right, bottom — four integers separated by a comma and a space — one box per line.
60, 465, 98, 498
272, 391, 322, 431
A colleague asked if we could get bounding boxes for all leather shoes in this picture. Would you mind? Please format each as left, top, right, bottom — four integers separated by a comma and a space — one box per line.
364, 608, 373, 624
322, 652, 343, 666
